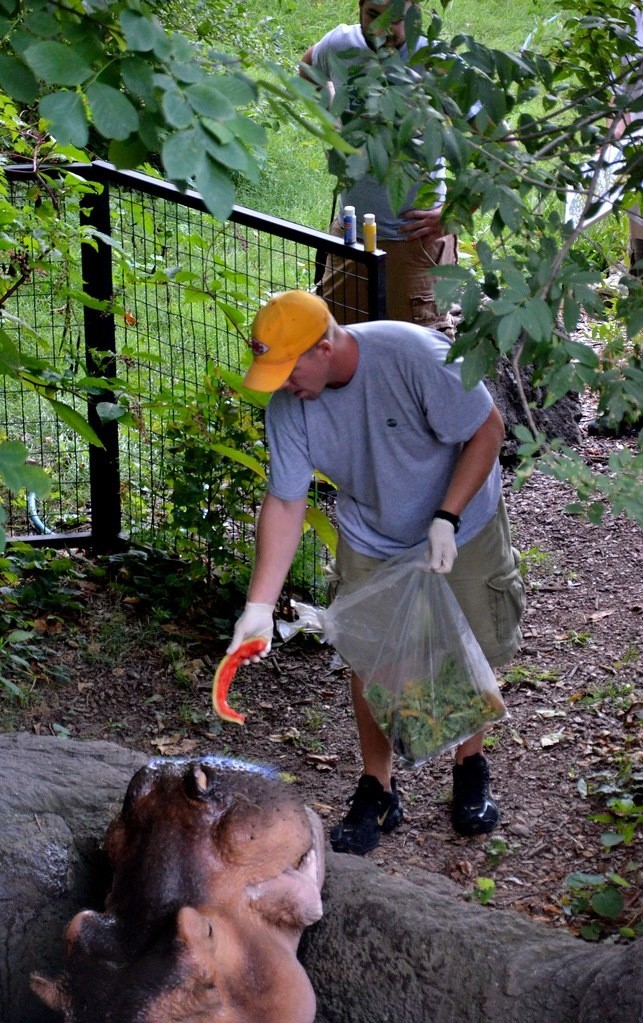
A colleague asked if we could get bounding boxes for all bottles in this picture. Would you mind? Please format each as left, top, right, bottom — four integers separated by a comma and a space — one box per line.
363, 213, 377, 252
343, 206, 356, 245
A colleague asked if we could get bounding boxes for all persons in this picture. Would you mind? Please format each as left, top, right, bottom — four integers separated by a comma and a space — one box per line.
597, 0, 643, 295
225, 290, 527, 854
298, 0, 520, 341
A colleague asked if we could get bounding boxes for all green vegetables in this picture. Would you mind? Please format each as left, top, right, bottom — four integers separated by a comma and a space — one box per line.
361, 648, 503, 760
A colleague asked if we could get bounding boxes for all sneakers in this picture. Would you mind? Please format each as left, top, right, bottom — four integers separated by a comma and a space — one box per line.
329, 774, 404, 856
449, 752, 499, 836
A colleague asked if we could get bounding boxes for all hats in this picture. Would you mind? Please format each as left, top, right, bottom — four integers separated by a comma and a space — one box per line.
241, 290, 329, 393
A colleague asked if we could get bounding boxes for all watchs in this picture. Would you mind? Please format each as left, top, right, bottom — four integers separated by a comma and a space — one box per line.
431, 509, 462, 534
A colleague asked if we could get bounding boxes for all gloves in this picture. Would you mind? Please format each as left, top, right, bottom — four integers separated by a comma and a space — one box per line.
422, 517, 458, 574
226, 602, 276, 666
